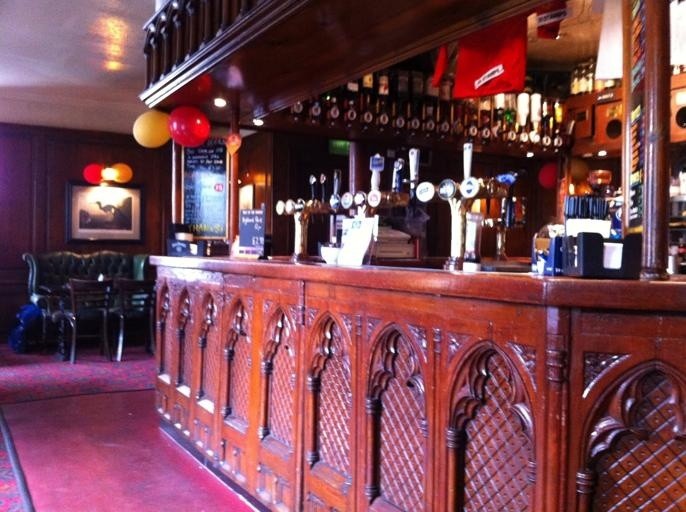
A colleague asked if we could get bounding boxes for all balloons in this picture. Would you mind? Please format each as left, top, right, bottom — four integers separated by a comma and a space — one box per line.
110, 163, 134, 183
131, 109, 173, 149
167, 107, 210, 146
82, 163, 103, 184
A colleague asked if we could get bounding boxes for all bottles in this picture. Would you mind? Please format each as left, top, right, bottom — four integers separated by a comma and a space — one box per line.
289, 72, 565, 159
569, 61, 616, 96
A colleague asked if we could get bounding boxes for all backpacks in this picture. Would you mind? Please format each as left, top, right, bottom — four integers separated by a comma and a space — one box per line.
8, 305, 42, 355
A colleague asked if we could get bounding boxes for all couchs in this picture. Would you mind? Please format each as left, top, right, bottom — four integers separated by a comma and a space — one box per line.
22, 248, 149, 354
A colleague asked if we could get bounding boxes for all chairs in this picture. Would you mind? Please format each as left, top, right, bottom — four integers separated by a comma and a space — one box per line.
46, 273, 155, 358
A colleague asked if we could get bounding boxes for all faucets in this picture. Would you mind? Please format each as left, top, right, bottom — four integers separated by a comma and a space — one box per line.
276, 145, 522, 262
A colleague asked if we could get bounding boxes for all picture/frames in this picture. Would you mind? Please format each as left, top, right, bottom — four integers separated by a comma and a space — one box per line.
66, 182, 142, 243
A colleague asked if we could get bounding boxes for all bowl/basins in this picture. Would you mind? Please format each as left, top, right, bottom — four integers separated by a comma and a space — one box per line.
319, 242, 345, 265
